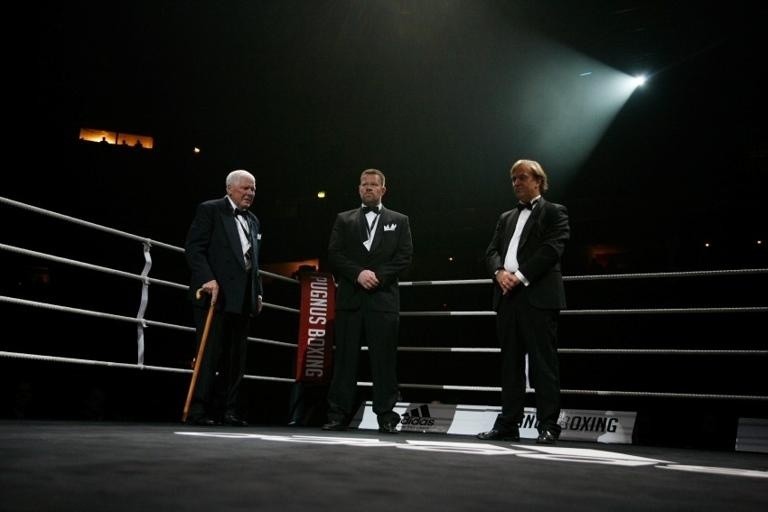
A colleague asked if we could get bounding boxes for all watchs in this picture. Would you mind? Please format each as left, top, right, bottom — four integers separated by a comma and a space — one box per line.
494, 268, 504, 281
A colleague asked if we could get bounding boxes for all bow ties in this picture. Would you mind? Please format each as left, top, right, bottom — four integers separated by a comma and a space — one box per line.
363, 206, 379, 215
518, 201, 532, 211
234, 208, 248, 216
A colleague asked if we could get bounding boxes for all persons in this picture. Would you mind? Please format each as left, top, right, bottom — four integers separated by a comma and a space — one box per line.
184, 170, 264, 426
476, 160, 571, 445
322, 169, 413, 434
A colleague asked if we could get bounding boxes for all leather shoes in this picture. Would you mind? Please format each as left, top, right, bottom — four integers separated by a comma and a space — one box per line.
379, 421, 400, 434
477, 427, 520, 442
188, 413, 249, 427
322, 418, 348, 431
536, 429, 558, 444
